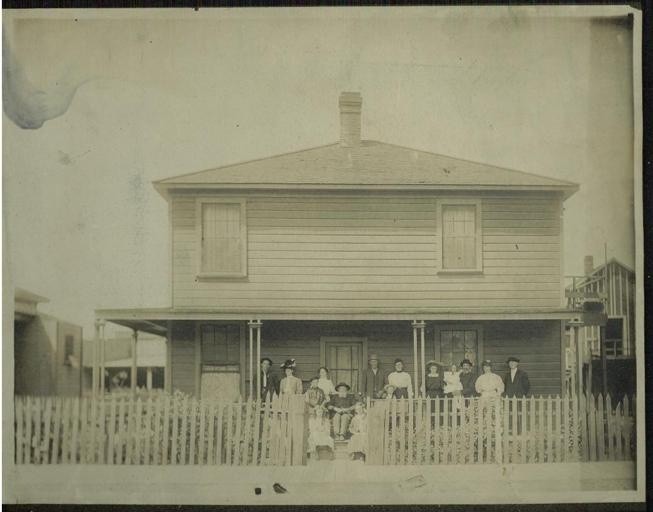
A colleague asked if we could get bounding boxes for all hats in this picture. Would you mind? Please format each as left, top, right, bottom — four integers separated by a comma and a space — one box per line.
279, 358, 296, 370
334, 381, 350, 392
506, 356, 518, 363
459, 358, 473, 367
367, 354, 379, 362
426, 361, 442, 372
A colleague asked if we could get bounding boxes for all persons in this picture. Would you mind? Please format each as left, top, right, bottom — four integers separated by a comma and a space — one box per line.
253, 354, 529, 463
